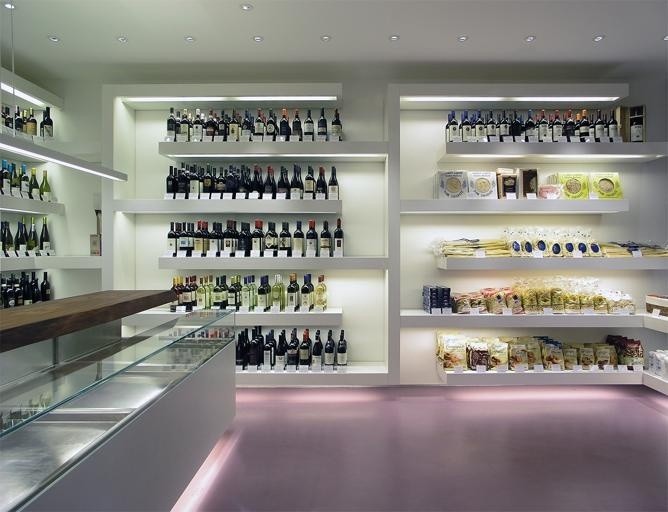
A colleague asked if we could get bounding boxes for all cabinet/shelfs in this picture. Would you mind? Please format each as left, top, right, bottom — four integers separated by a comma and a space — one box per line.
0, 66, 129, 309
100, 82, 668, 397
0, 289, 237, 512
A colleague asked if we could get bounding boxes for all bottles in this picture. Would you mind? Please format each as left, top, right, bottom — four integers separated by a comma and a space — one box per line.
318, 108, 327, 141
462, 110, 472, 141
548, 114, 554, 129
26, 108, 37, 136
206, 107, 216, 142
194, 108, 203, 143
249, 326, 264, 365
218, 110, 227, 141
166, 166, 175, 199
236, 328, 248, 370
190, 163, 200, 199
279, 222, 290, 257
525, 109, 535, 143
229, 107, 239, 141
242, 109, 252, 141
320, 220, 331, 256
224, 220, 233, 257
250, 164, 261, 199
201, 113, 206, 137
40, 170, 51, 201
204, 276, 211, 309
30, 271, 40, 301
271, 273, 285, 312
228, 277, 237, 311
237, 275, 242, 306
168, 222, 177, 256
183, 277, 192, 311
234, 276, 240, 310
226, 164, 235, 200
20, 165, 29, 197
534, 113, 541, 131
575, 114, 581, 136
299, 329, 312, 366
14, 105, 23, 131
445, 114, 451, 141
177, 276, 183, 306
216, 166, 226, 193
43, 107, 53, 137
475, 110, 484, 143
179, 222, 189, 257
278, 167, 288, 198
189, 113, 194, 142
193, 275, 198, 290
287, 272, 299, 312
23, 275, 33, 304
315, 274, 327, 312
208, 275, 214, 305
247, 276, 254, 310
40, 217, 50, 255
233, 221, 239, 249
305, 166, 314, 200
484, 114, 489, 136
263, 330, 276, 370
194, 220, 208, 257
316, 167, 326, 199
487, 110, 496, 142
312, 330, 323, 365
496, 114, 502, 136
324, 330, 335, 365
21, 215, 28, 243
511, 110, 521, 135
28, 217, 39, 254
177, 161, 187, 199
251, 275, 258, 306
251, 220, 261, 257
254, 108, 264, 136
209, 222, 224, 257
292, 109, 302, 141
603, 114, 608, 137
241, 277, 250, 312
589, 114, 594, 136
328, 166, 338, 200
608, 110, 617, 142
40, 111, 46, 137
238, 222, 247, 256
539, 111, 549, 142
565, 110, 576, 142
334, 218, 344, 256
29, 168, 39, 199
580, 109, 590, 142
4, 279, 15, 308
212, 168, 217, 193
332, 108, 342, 141
214, 277, 222, 309
176, 111, 181, 133
168, 328, 235, 367
14, 280, 23, 305
175, 222, 180, 251
10, 164, 19, 196
265, 221, 274, 257
301, 274, 314, 311
450, 110, 458, 141
189, 276, 197, 306
290, 164, 300, 199
263, 166, 274, 199
287, 327, 299, 365
187, 223, 194, 257
275, 330, 287, 369
306, 220, 318, 256
294, 221, 304, 257
552, 110, 562, 142
266, 108, 276, 141
2, 221, 13, 257
181, 276, 184, 288
15, 221, 26, 256
203, 163, 212, 193
170, 278, 178, 311
0, 160, 10, 195
41, 272, 50, 301
223, 275, 228, 306
280, 109, 289, 141
337, 330, 348, 366
594, 110, 604, 142
196, 278, 206, 309
304, 110, 314, 141
238, 164, 248, 198
167, 107, 175, 141
499, 110, 509, 141
220, 276, 226, 309
181, 109, 190, 142
459, 114, 464, 142
257, 275, 271, 312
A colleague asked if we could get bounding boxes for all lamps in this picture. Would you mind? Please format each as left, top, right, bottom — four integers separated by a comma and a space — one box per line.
238, 4, 254, 13
4, 2, 15, 13
